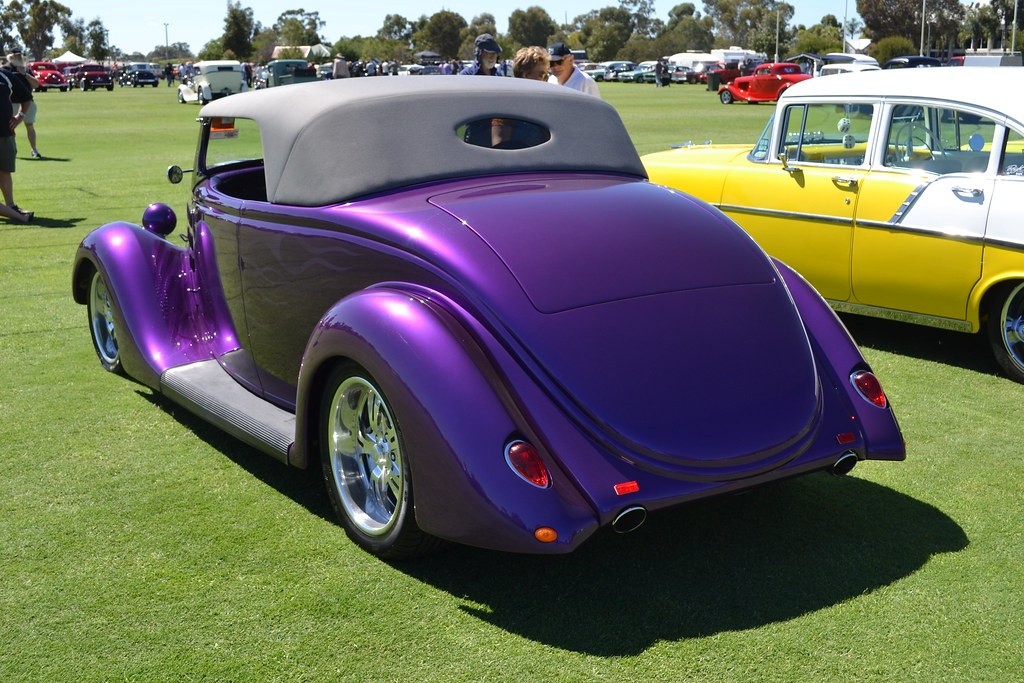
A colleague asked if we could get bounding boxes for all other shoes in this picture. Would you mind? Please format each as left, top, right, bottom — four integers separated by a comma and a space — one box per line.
31, 150, 41, 159
13, 205, 34, 219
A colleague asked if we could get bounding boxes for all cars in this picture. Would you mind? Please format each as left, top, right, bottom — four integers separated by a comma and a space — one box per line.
390, 64, 424, 75
579, 61, 721, 84
148, 63, 163, 79
58, 64, 113, 91
70, 74, 905, 559
172, 64, 179, 78
25, 62, 68, 92
178, 61, 250, 104
716, 62, 812, 105
118, 62, 158, 87
819, 53, 1023, 123
320, 63, 335, 80
640, 69, 1024, 381
699, 63, 740, 84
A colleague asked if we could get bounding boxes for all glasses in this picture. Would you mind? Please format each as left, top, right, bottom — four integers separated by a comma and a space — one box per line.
550, 59, 564, 67
529, 71, 551, 79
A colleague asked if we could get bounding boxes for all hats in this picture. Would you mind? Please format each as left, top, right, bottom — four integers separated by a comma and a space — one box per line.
10, 48, 24, 55
548, 43, 571, 61
335, 52, 345, 59
475, 34, 503, 53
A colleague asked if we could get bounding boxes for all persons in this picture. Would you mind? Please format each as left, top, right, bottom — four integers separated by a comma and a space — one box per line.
740, 60, 749, 75
111, 53, 463, 90
0, 46, 42, 158
458, 34, 599, 150
0, 68, 37, 222
799, 59, 813, 76
655, 58, 670, 87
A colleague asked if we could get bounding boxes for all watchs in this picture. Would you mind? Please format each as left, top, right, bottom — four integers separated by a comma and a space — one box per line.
18, 111, 25, 117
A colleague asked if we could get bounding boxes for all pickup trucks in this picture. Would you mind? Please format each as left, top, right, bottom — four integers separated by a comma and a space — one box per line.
255, 60, 323, 89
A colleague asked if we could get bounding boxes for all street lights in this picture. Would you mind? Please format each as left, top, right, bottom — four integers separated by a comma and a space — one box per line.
164, 23, 169, 60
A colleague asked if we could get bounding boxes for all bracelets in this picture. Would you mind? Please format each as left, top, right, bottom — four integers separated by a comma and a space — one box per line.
24, 72, 26, 76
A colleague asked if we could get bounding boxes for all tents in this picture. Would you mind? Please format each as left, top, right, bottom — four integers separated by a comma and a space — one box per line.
52, 51, 87, 64
787, 53, 855, 78
415, 50, 440, 62
